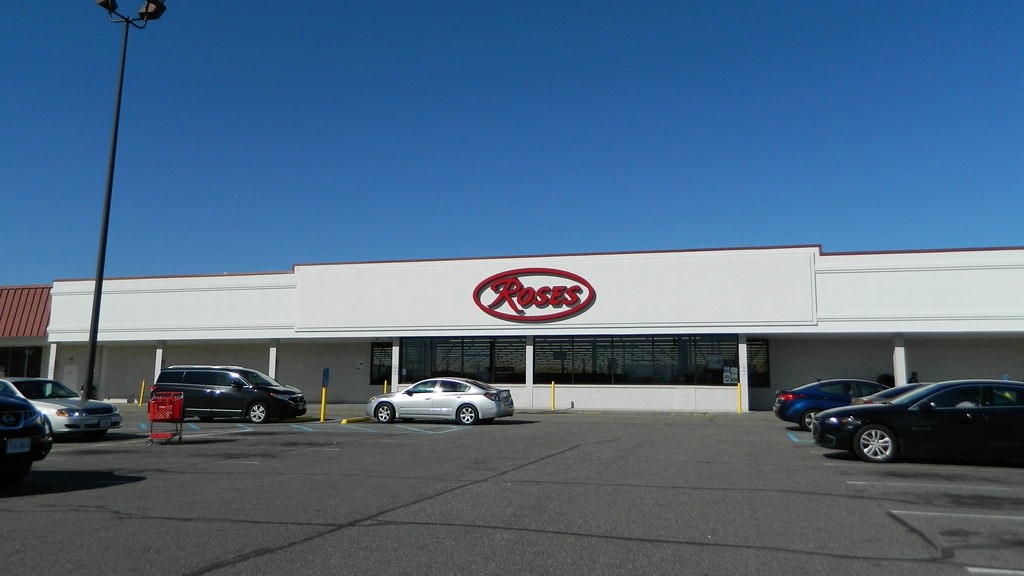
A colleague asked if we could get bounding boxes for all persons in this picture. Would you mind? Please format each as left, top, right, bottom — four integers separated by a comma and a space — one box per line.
908, 371, 918, 383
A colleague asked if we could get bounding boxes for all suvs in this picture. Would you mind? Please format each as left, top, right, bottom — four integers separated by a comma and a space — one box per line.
148, 363, 306, 423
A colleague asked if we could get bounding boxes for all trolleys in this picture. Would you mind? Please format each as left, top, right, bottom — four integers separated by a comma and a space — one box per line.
146, 391, 184, 445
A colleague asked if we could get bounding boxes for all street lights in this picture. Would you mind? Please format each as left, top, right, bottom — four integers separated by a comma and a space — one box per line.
80, 2, 171, 395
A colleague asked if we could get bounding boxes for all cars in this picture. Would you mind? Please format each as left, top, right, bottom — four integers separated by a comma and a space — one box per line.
849, 382, 932, 408
0, 378, 52, 486
366, 377, 515, 427
808, 380, 1023, 465
774, 380, 892, 432
4, 377, 122, 440
